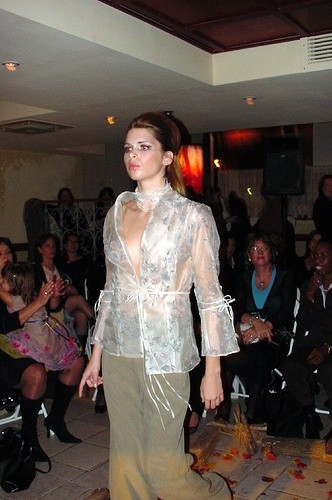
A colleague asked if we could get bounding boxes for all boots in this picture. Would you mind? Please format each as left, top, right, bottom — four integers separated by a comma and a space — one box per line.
20, 395, 51, 462
45, 382, 82, 443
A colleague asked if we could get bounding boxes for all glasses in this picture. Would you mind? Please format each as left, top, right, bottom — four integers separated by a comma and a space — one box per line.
251, 245, 268, 253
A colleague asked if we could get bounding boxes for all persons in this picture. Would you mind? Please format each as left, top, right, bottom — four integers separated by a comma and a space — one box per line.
187, 175, 332, 454
78, 112, 241, 500
0, 187, 115, 462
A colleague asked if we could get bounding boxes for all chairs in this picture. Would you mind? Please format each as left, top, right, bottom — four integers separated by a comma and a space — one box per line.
0, 402, 56, 436
203, 288, 299, 417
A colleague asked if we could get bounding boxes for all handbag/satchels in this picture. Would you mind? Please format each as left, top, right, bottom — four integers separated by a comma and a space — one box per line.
0, 426, 51, 493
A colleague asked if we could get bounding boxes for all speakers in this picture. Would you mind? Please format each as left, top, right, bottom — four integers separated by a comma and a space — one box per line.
264, 135, 304, 195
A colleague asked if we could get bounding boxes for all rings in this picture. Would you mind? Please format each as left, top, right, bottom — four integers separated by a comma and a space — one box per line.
318, 281, 319, 282
45, 293, 47, 294
251, 335, 252, 337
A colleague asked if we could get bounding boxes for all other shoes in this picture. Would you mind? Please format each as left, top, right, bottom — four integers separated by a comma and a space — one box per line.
214, 400, 232, 423
188, 416, 200, 434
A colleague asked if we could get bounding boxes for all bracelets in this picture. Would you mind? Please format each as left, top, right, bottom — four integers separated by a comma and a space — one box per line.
327, 345, 330, 355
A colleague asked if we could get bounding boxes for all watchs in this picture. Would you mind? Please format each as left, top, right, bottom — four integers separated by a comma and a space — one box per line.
249, 313, 255, 324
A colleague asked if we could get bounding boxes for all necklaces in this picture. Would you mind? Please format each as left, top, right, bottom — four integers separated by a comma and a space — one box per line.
259, 281, 264, 286
